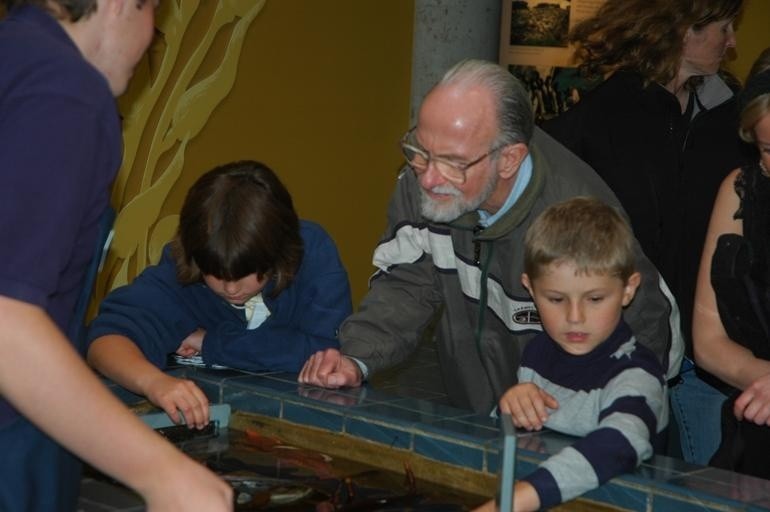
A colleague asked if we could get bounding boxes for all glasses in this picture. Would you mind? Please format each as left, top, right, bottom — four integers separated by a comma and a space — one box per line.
399, 125, 509, 184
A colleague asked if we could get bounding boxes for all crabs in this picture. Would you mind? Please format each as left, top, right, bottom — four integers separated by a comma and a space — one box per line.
224, 467, 268, 483
244, 481, 314, 507
242, 425, 278, 451
275, 446, 337, 481
346, 462, 421, 505
316, 484, 341, 512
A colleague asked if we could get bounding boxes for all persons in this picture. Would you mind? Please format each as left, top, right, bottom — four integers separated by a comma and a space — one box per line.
79, 158, 355, 434
691, 48, 770, 484
537, 0, 753, 296
293, 58, 690, 432
464, 192, 672, 510
2, 1, 238, 512
